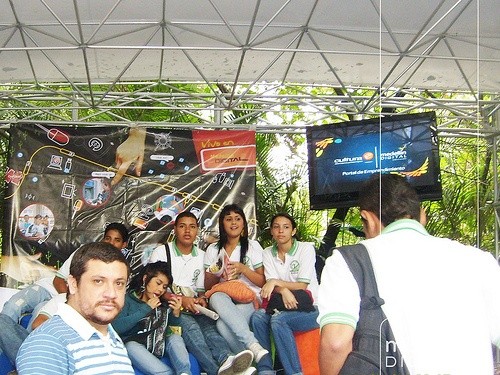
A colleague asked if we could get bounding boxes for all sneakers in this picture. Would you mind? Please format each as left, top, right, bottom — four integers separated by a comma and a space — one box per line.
240, 367, 256, 375
247, 342, 269, 364
217, 350, 253, 375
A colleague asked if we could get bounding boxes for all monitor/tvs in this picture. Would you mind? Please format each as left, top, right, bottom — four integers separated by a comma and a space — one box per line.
308, 111, 442, 209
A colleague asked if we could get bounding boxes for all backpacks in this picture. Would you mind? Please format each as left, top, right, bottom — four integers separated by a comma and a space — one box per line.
123, 306, 168, 359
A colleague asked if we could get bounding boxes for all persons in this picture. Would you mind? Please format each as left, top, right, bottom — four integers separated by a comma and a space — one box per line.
19, 215, 30, 235
13, 241, 136, 375
86, 182, 109, 205
313, 170, 499, 375
202, 202, 269, 375
40, 215, 50, 236
52, 222, 130, 294
30, 290, 70, 329
24, 215, 44, 241
109, 260, 182, 375
248, 214, 320, 375
149, 211, 258, 375
110, 128, 146, 185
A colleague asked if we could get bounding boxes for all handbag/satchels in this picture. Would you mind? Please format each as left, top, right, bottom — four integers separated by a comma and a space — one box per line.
328, 244, 410, 375
266, 290, 316, 313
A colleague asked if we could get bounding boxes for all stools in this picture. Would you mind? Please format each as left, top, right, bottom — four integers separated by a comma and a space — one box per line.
248, 325, 276, 371
132, 351, 202, 375
1, 314, 33, 374
294, 329, 323, 374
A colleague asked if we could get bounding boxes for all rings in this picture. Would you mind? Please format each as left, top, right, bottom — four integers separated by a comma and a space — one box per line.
233, 269, 236, 271
